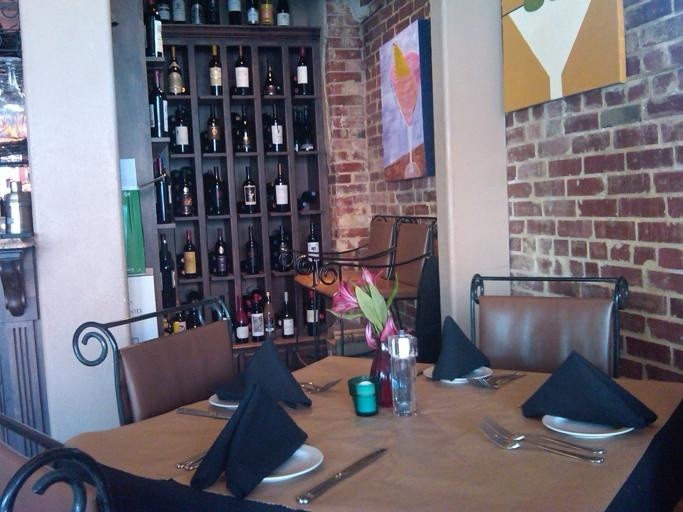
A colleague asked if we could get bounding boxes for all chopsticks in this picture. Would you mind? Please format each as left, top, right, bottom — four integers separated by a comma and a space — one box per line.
173, 435, 206, 472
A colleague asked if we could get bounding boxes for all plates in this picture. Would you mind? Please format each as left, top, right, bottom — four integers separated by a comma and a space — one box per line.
208, 393, 242, 410
422, 365, 495, 386
541, 413, 635, 440
260, 442, 324, 483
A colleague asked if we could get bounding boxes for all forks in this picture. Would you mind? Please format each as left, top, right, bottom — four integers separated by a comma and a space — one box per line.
484, 416, 608, 455
298, 378, 342, 394
469, 365, 527, 392
477, 422, 606, 464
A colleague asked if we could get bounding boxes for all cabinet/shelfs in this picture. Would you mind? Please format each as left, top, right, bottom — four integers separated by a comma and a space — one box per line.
0, 241, 53, 458
143, 25, 335, 373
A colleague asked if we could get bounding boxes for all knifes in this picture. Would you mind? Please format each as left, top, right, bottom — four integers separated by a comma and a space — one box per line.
295, 447, 393, 506
176, 406, 234, 420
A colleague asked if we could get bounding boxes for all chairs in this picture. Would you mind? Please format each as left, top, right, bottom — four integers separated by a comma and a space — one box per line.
73, 296, 238, 427
302, 217, 446, 362
469, 273, 630, 378
0, 413, 110, 512
281, 216, 397, 359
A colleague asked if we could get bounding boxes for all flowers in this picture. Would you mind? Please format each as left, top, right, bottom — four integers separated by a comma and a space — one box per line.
327, 259, 400, 350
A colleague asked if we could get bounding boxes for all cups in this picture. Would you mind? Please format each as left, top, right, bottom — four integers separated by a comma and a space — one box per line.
347, 374, 382, 417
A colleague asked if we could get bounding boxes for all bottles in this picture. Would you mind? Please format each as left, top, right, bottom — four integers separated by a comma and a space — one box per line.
172, 1, 186, 23
167, 46, 183, 95
216, 296, 225, 319
237, 104, 254, 153
273, 164, 289, 212
183, 229, 196, 278
214, 228, 228, 277
234, 296, 250, 343
209, 45, 223, 96
267, 104, 284, 152
233, 46, 250, 96
276, 1, 290, 27
154, 158, 173, 224
281, 291, 295, 338
299, 107, 313, 151
305, 290, 320, 337
260, 0, 274, 26
264, 55, 279, 96
190, 307, 200, 328
249, 291, 264, 342
228, 0, 242, 25
205, 0, 219, 25
149, 69, 169, 138
263, 292, 275, 339
5, 182, 33, 238
160, 234, 177, 308
172, 103, 190, 154
294, 47, 310, 95
157, 0, 171, 22
208, 102, 221, 153
306, 218, 321, 265
210, 166, 225, 216
178, 168, 192, 216
173, 311, 186, 333
144, 1, 164, 57
243, 166, 257, 213
278, 226, 289, 271
389, 331, 418, 418
191, 1, 205, 25
246, 226, 258, 274
247, 0, 259, 26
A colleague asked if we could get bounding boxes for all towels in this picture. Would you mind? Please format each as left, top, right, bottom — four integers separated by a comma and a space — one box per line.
432, 316, 490, 381
217, 336, 313, 408
521, 351, 658, 428
191, 385, 308, 497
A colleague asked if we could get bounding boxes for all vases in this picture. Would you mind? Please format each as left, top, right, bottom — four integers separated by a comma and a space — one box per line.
370, 342, 391, 409
